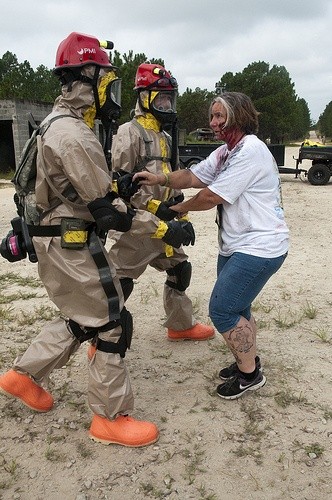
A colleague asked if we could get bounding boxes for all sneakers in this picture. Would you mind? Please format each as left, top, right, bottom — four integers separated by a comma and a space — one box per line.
217, 369, 267, 399
219, 356, 261, 380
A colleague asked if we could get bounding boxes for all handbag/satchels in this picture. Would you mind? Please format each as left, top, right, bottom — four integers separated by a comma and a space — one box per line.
10, 112, 84, 225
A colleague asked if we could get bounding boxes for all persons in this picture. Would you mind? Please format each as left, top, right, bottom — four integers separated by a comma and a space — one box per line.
87, 63, 216, 361
0, 31, 195, 448
132, 91, 291, 401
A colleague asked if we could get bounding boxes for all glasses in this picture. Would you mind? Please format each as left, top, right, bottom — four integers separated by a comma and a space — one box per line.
141, 77, 178, 87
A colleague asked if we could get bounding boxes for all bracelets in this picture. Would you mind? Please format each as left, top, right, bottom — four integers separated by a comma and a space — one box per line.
159, 172, 169, 187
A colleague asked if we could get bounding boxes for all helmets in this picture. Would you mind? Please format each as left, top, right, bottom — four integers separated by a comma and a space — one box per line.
52, 30, 114, 75
133, 63, 178, 91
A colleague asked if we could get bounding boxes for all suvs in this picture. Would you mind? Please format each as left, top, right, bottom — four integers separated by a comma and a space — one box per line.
296, 142, 332, 185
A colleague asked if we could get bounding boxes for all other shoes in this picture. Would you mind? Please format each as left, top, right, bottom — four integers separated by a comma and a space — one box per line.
0, 368, 54, 413
87, 343, 97, 362
89, 413, 159, 447
168, 323, 215, 341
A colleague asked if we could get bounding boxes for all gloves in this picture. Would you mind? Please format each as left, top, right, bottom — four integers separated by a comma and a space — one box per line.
147, 193, 184, 222
110, 173, 145, 205
150, 214, 195, 248
